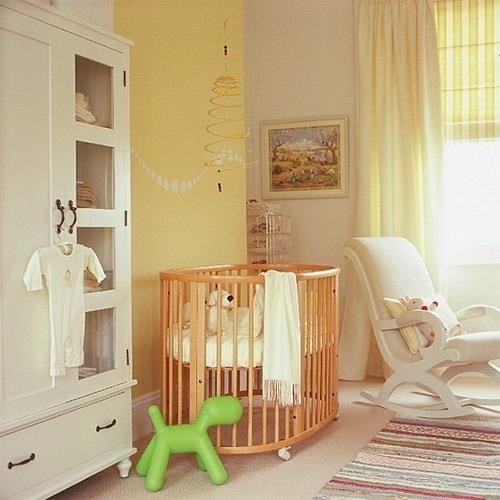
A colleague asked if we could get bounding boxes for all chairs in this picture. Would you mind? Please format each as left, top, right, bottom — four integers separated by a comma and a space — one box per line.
342, 237, 500, 419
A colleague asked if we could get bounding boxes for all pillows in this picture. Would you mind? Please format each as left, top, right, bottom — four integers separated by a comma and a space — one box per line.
382, 295, 462, 354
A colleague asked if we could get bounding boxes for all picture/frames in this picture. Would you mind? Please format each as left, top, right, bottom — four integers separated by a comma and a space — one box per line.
261, 114, 347, 200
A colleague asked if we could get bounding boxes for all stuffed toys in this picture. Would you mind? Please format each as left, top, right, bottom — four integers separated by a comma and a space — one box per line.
398, 296, 459, 348
182, 289, 232, 339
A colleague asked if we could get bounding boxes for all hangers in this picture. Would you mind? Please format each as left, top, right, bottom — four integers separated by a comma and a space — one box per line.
56, 224, 72, 246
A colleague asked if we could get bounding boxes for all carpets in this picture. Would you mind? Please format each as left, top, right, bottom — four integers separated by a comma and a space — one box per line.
311, 414, 500, 500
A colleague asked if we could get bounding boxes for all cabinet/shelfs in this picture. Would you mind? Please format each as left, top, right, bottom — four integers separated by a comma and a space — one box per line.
2, 0, 138, 500
